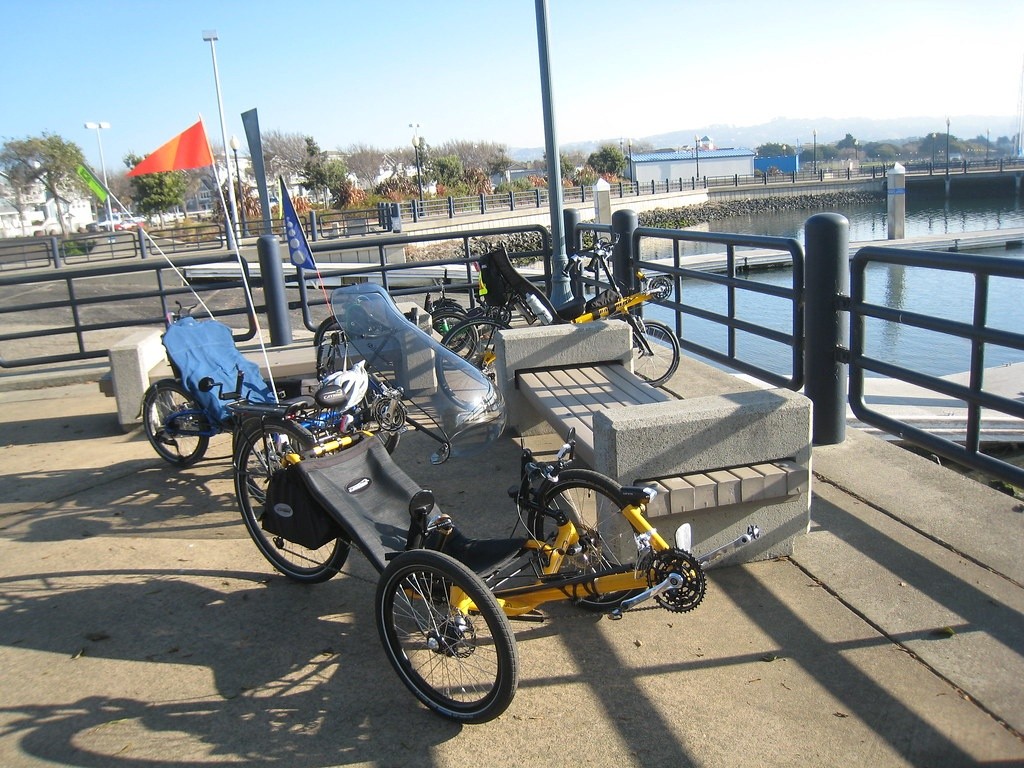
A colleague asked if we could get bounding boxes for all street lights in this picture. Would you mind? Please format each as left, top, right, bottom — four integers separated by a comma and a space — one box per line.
694, 135, 700, 181
204, 29, 244, 249
412, 133, 427, 217
229, 134, 252, 238
85, 122, 116, 233
946, 118, 950, 174
812, 130, 818, 174
931, 133, 937, 170
627, 137, 635, 194
854, 139, 859, 160
984, 128, 990, 161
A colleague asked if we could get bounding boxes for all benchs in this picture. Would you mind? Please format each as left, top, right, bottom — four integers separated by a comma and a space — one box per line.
99, 300, 436, 434
494, 319, 814, 564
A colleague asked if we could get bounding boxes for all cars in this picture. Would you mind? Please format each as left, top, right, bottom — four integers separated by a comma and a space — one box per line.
96, 209, 190, 233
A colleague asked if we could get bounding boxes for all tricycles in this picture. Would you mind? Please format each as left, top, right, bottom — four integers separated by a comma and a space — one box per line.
232, 281, 760, 725
136, 225, 682, 469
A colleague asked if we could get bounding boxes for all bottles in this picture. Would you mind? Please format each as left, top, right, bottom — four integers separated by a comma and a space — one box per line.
525, 293, 554, 326
442, 318, 450, 339
425, 513, 453, 554
